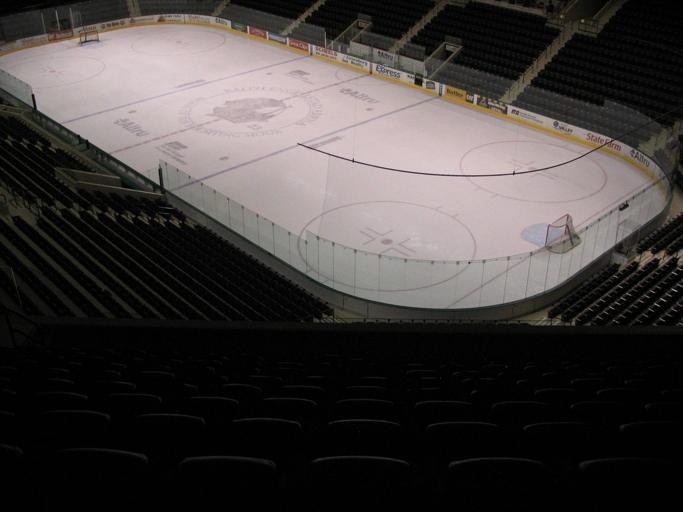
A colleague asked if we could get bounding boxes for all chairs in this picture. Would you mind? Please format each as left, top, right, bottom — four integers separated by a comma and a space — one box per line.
0, 0, 683, 512
